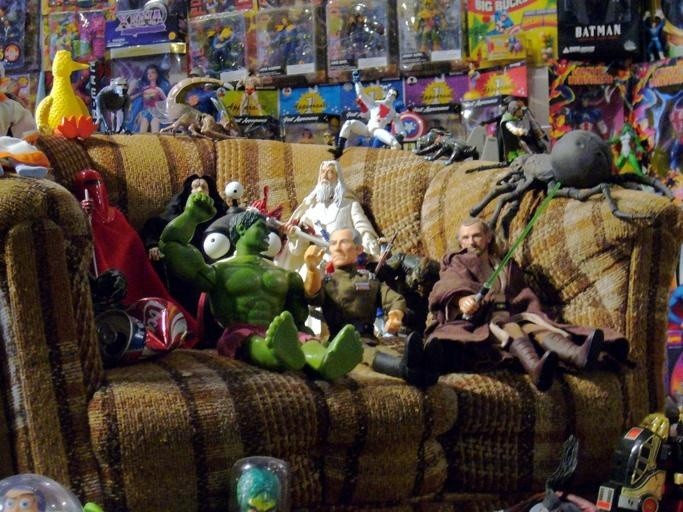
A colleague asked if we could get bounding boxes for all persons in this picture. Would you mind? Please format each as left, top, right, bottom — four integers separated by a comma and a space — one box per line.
0, 1, 682, 390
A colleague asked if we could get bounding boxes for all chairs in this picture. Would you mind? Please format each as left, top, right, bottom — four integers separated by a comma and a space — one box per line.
1, 134, 683, 511
